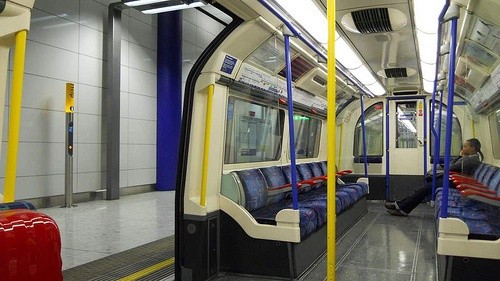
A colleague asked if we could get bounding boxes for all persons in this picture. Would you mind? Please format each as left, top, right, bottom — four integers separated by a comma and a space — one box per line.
385, 138, 481, 216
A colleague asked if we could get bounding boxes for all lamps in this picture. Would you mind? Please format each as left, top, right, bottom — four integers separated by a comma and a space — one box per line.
411, 0, 451, 93
273, 1, 387, 97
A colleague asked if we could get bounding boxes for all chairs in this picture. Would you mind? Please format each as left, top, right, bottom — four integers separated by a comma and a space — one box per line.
434, 160, 500, 281
218, 160, 370, 280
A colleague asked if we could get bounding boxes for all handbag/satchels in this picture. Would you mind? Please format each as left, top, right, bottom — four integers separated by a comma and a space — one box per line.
426, 164, 444, 183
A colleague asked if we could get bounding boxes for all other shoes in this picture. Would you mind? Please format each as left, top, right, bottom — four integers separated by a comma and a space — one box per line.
385, 202, 397, 210
385, 209, 403, 216
